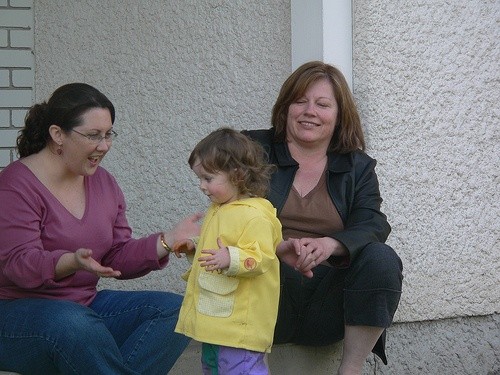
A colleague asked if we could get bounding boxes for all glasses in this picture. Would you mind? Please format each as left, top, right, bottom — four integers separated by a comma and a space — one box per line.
70, 128, 118, 143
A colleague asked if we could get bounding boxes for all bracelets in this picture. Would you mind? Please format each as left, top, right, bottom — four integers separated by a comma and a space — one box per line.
160, 232, 172, 252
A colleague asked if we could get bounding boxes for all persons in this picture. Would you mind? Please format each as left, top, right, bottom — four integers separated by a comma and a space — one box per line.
240, 61, 404, 375
172, 127, 282, 375
0, 83, 206, 375
